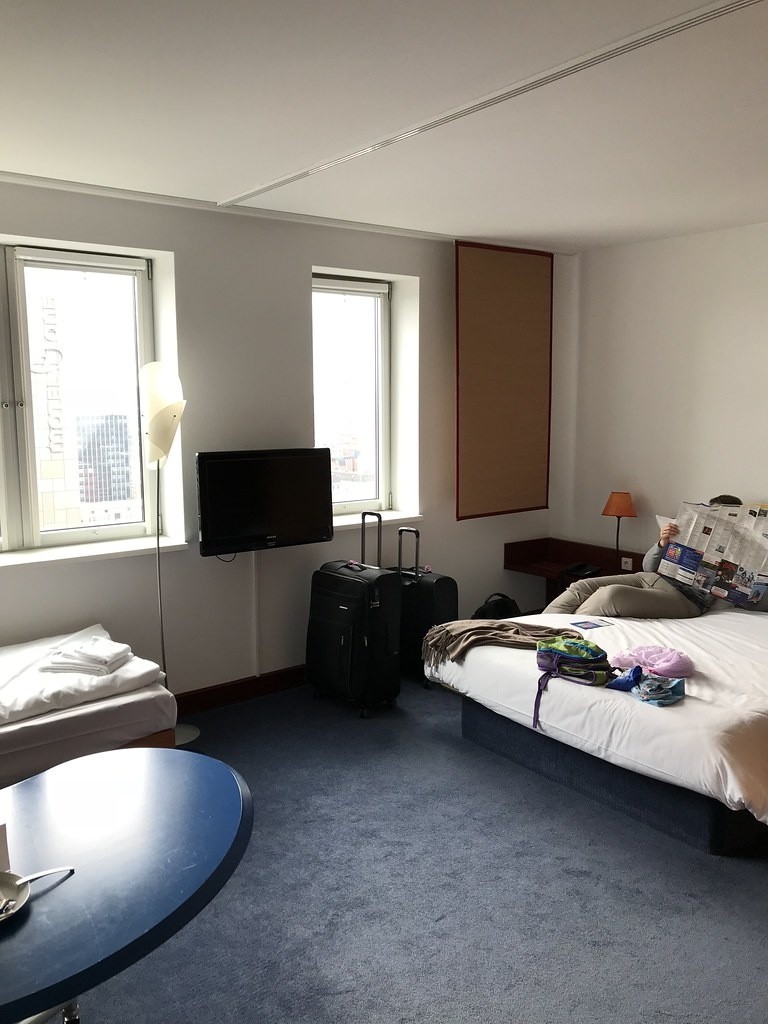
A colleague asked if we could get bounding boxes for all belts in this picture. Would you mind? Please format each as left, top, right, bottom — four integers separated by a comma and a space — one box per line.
657, 572, 709, 612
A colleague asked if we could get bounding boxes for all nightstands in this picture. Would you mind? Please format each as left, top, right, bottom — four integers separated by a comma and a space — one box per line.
503, 536, 645, 608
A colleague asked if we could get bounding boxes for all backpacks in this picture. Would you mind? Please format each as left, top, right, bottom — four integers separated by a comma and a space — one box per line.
532, 636, 626, 727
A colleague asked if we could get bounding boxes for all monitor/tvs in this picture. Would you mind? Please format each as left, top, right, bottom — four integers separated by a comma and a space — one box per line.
196, 447, 334, 557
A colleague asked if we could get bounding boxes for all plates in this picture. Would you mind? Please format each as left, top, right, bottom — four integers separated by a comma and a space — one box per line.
0, 872, 30, 921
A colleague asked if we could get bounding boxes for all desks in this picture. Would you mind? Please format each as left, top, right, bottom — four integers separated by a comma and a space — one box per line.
0, 747, 253, 1024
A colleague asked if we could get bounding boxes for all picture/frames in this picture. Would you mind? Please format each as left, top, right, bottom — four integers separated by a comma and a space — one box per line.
454, 239, 555, 524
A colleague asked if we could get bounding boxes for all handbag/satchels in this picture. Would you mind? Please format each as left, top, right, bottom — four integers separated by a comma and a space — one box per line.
470, 592, 521, 619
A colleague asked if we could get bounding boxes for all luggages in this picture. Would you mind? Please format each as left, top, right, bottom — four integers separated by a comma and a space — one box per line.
306, 512, 403, 718
387, 526, 458, 690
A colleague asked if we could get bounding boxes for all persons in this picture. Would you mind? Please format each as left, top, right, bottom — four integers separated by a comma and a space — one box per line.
541, 495, 744, 619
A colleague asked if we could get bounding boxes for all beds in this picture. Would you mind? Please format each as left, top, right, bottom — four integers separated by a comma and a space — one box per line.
0, 622, 178, 791
422, 598, 768, 855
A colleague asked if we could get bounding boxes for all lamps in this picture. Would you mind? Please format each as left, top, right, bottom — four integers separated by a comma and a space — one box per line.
601, 492, 639, 551
138, 360, 201, 748
